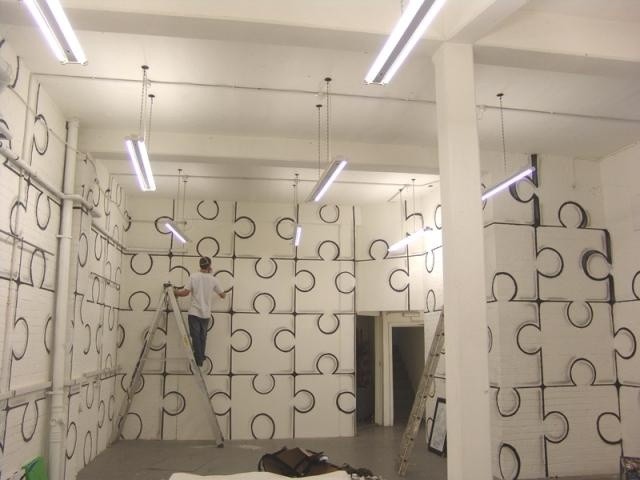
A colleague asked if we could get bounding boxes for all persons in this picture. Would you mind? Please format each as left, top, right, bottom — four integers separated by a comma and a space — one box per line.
174, 256, 226, 367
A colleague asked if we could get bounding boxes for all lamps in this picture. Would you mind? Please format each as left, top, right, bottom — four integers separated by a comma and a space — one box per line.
164, 169, 188, 244
366, 2, 441, 84
124, 65, 157, 193
19, 0, 86, 65
293, 173, 302, 247
481, 93, 535, 200
309, 78, 348, 203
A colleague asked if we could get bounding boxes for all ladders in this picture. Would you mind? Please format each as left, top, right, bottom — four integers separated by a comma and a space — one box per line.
105, 283, 224, 445
392, 306, 444, 477
